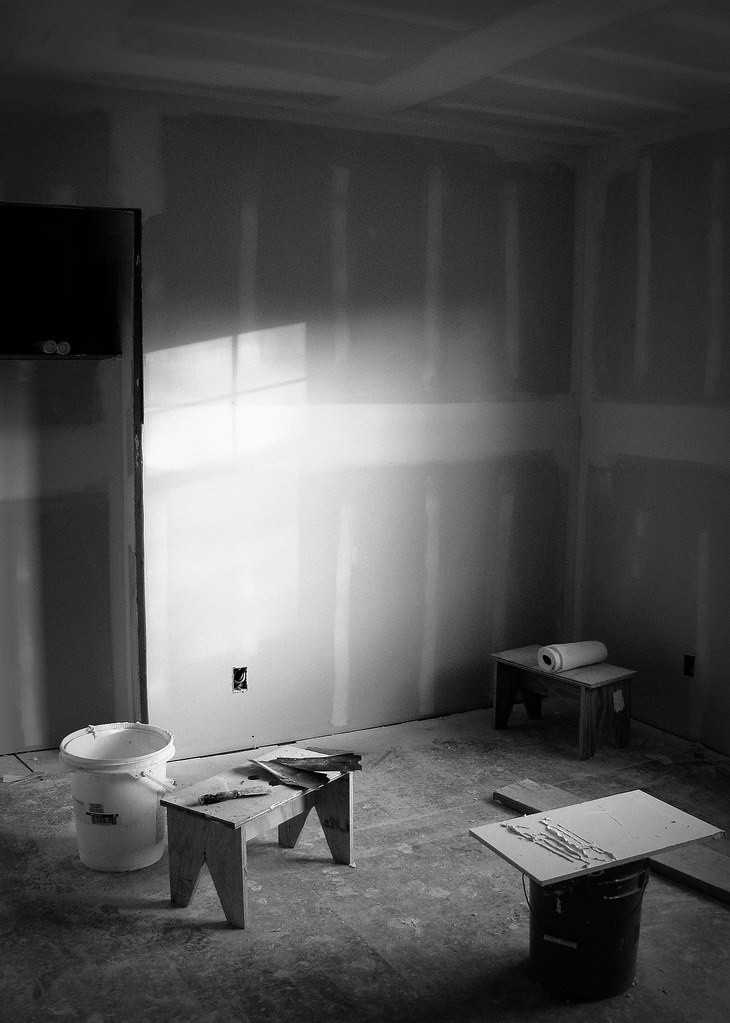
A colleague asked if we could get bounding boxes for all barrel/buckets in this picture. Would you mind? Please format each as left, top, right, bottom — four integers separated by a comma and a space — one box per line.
57, 721, 176, 874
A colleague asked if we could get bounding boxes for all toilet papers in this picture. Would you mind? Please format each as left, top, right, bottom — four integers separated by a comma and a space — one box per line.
537, 641, 607, 674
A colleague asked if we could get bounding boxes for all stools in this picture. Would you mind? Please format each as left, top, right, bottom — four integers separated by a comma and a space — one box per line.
490, 644, 637, 762
159, 744, 354, 929
